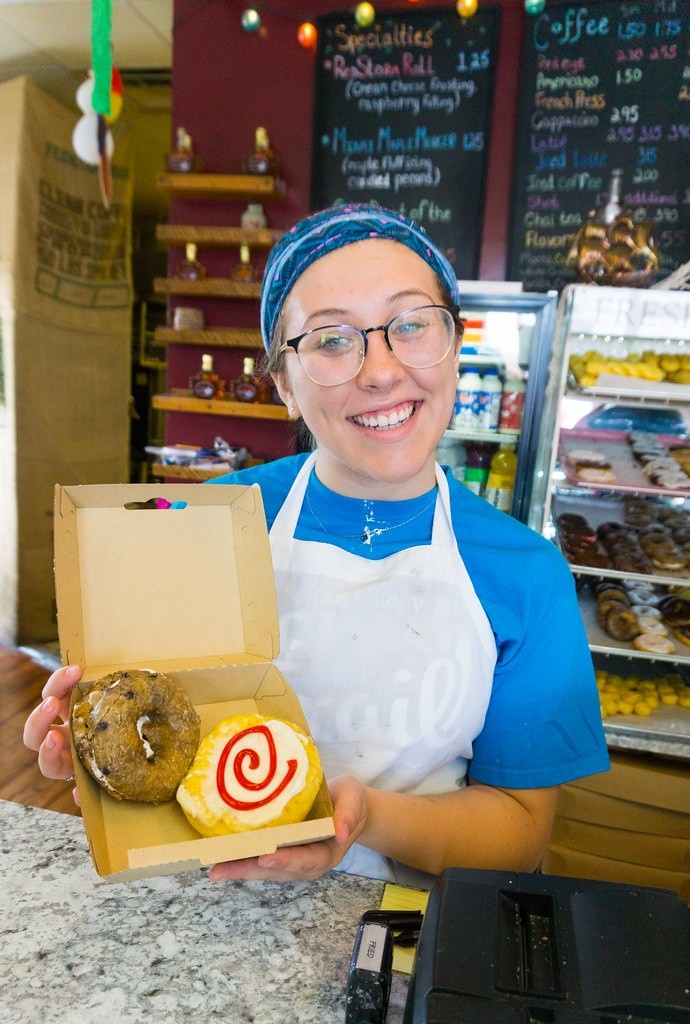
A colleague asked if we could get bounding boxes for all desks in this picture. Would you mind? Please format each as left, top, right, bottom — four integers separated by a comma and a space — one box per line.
0, 799, 429, 1024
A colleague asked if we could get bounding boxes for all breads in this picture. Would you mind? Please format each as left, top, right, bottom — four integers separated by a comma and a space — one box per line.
570, 347, 690, 390
595, 668, 690, 718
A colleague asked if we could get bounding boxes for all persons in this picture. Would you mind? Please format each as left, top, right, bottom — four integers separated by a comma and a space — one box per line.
22, 202, 611, 890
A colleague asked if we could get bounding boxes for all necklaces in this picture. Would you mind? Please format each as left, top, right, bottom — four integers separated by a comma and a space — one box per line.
307, 487, 436, 537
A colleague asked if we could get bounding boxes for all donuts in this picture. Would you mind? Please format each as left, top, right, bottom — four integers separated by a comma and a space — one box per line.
569, 433, 690, 490
593, 578, 690, 656
72, 670, 201, 805
559, 500, 690, 579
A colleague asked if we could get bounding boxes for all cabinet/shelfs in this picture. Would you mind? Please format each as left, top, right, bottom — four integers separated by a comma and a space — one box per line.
151, 172, 300, 479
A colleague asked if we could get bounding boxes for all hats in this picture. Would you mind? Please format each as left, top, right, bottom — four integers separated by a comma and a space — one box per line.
260, 202, 461, 358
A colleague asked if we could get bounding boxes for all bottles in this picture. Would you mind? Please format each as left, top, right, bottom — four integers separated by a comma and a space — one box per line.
240, 202, 267, 228
173, 242, 206, 278
242, 127, 283, 176
435, 437, 517, 520
450, 368, 525, 435
269, 375, 286, 406
231, 357, 265, 404
232, 244, 260, 281
189, 354, 225, 400
167, 128, 205, 173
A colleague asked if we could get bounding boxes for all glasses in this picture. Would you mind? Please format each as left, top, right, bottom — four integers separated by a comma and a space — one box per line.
280, 305, 460, 388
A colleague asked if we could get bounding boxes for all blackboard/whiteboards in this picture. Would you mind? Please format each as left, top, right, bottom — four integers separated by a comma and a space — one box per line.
310, 13, 499, 281
507, 1, 690, 290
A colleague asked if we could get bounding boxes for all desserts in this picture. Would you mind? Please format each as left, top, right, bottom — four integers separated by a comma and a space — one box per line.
176, 715, 324, 837
